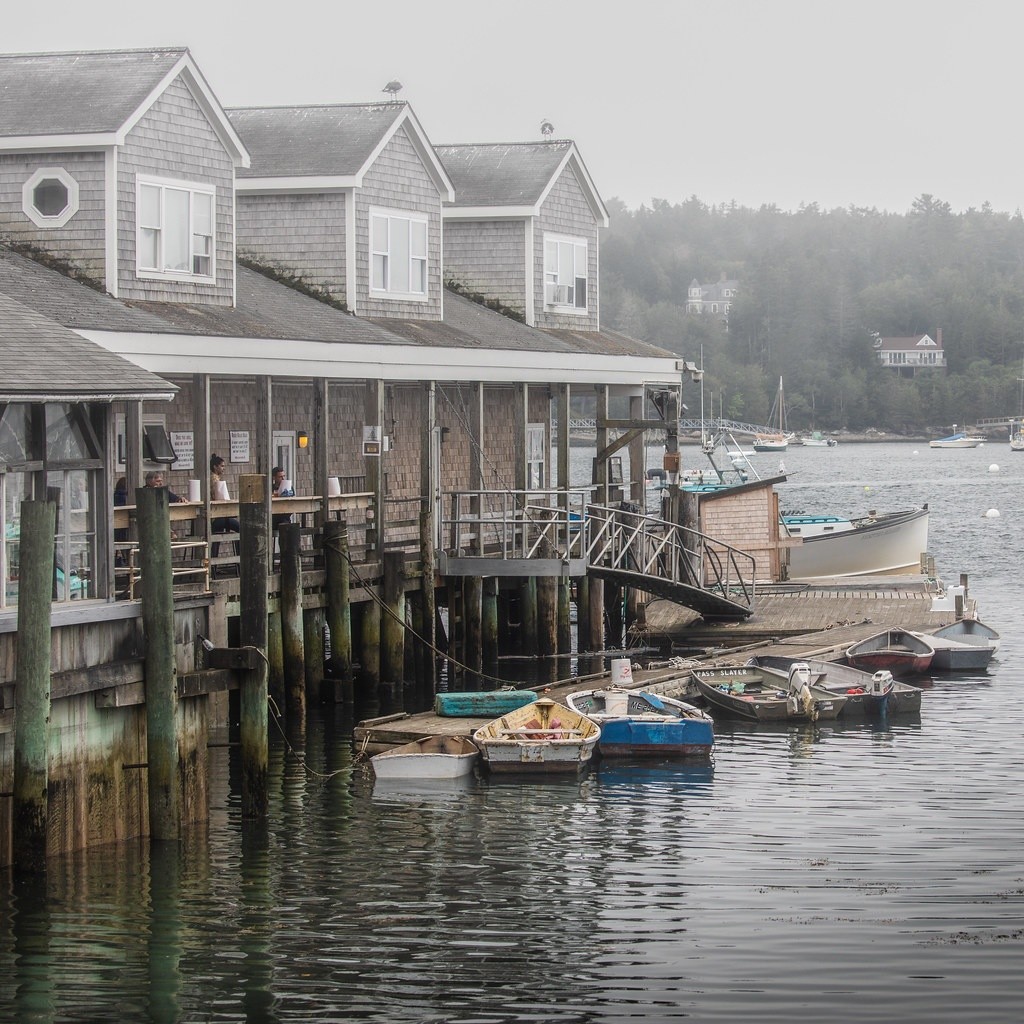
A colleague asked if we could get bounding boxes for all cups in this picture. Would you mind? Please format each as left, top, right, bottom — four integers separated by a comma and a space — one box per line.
274, 490, 279, 497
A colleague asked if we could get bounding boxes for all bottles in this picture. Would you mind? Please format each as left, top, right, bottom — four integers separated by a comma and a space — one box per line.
198, 635, 215, 651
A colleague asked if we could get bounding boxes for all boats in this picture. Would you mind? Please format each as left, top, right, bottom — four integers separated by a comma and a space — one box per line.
688, 665, 849, 723
929, 435, 987, 449
910, 630, 997, 672
476, 696, 604, 765
800, 437, 838, 448
1010, 432, 1024, 450
774, 503, 934, 578
367, 735, 480, 780
747, 654, 924, 712
845, 626, 935, 675
565, 686, 717, 757
932, 618, 1002, 653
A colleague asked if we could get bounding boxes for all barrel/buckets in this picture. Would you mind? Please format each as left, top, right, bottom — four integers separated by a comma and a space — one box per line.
605, 694, 629, 716
611, 659, 633, 685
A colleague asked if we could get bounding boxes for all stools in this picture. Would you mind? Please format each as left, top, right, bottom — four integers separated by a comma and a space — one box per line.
211, 531, 239, 579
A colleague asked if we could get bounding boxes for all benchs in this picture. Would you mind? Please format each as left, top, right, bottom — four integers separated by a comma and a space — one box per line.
501, 729, 581, 735
814, 682, 866, 691
728, 690, 782, 697
807, 671, 827, 676
700, 675, 763, 685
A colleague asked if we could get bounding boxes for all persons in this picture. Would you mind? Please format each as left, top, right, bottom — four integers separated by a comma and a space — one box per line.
110, 475, 127, 562
210, 452, 240, 570
271, 467, 294, 529
144, 470, 189, 503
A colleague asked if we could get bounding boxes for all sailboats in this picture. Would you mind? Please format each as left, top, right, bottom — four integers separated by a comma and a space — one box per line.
751, 375, 791, 451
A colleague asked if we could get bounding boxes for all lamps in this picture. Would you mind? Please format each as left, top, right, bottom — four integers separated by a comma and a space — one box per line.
441, 426, 450, 441
297, 431, 309, 448
